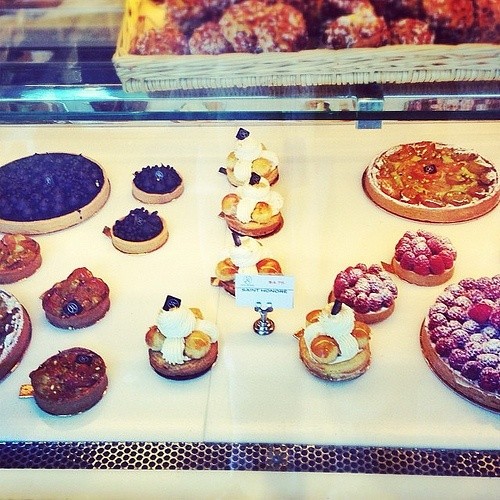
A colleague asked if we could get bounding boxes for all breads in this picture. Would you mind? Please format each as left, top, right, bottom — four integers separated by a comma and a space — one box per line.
127, 0, 500, 55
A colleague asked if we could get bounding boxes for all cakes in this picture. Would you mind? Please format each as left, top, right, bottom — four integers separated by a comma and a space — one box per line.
363, 141, 500, 222
132, 163, 183, 204
328, 263, 398, 324
0, 233, 110, 416
420, 274, 500, 414
110, 206, 169, 253
392, 230, 458, 287
0, 152, 110, 235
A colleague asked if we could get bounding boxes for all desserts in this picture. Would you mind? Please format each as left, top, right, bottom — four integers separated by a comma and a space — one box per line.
298, 299, 372, 381
145, 295, 218, 381
214, 127, 284, 300
